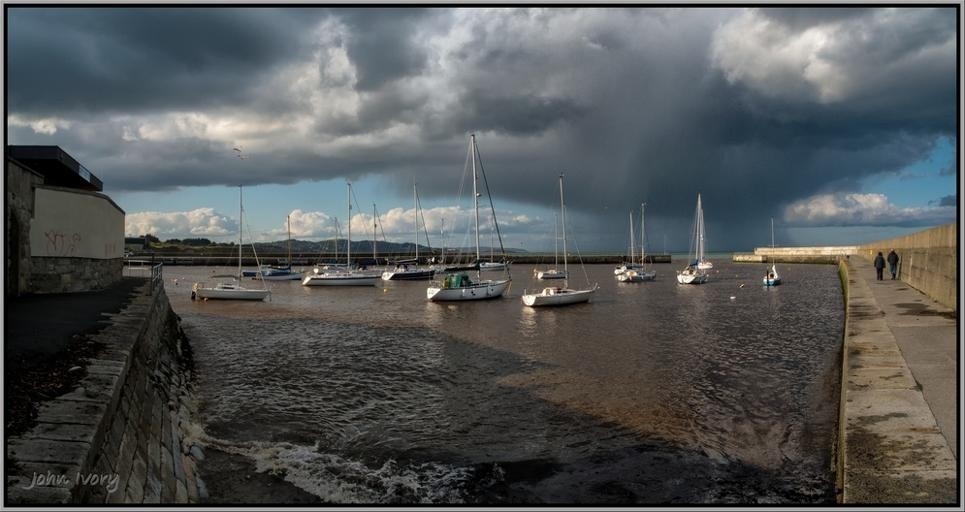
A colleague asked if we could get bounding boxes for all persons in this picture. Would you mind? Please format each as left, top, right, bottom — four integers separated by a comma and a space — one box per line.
874, 251, 886, 281
887, 248, 899, 280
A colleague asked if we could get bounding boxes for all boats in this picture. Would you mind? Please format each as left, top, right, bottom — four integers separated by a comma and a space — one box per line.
613, 202, 656, 283
425, 132, 512, 304
382, 184, 434, 281
762, 216, 781, 285
303, 182, 393, 286
676, 193, 713, 284
252, 215, 303, 280
521, 173, 598, 307
195, 183, 270, 301
